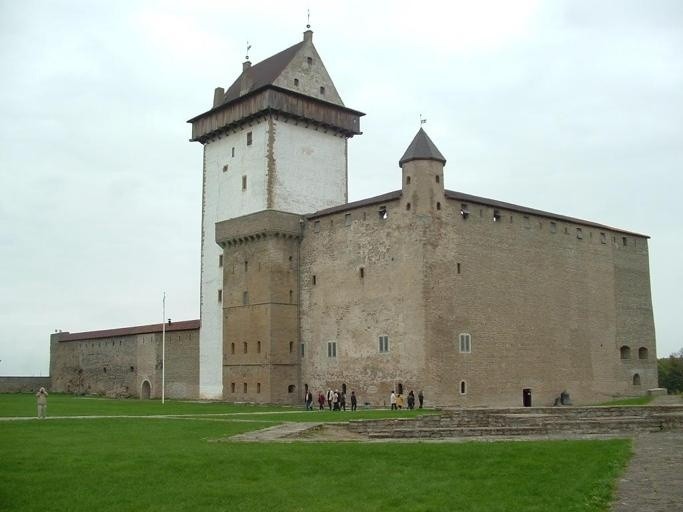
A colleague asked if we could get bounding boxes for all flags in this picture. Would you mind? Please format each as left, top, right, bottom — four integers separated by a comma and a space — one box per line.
421, 120, 426, 123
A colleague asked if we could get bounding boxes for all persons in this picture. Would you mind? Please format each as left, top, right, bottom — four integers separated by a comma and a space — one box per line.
303, 388, 357, 412
35, 386, 48, 419
389, 389, 424, 410
